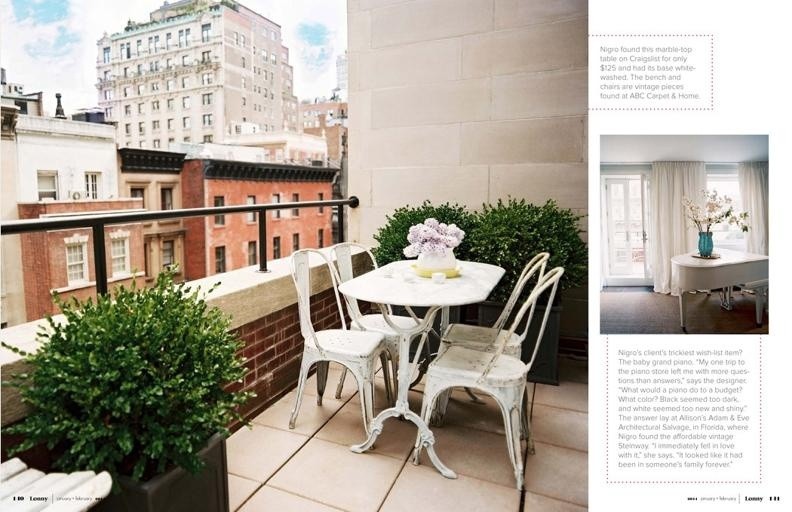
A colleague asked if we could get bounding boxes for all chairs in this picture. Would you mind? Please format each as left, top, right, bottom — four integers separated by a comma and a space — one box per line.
413, 252, 565, 491
290, 242, 430, 449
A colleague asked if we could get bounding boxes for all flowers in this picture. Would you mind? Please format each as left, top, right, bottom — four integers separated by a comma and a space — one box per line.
681, 188, 752, 250
403, 218, 466, 257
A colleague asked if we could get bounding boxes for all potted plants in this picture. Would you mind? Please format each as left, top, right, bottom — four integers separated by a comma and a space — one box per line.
368, 200, 471, 362
0, 263, 258, 510
469, 194, 587, 386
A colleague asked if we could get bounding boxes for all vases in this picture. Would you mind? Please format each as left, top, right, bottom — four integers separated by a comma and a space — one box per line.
698, 232, 713, 257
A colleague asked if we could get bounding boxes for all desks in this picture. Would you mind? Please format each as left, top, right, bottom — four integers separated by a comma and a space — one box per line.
671, 247, 769, 328
337, 259, 506, 480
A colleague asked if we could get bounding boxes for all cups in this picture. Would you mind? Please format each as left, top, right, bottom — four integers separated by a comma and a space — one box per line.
433, 274, 445, 286
